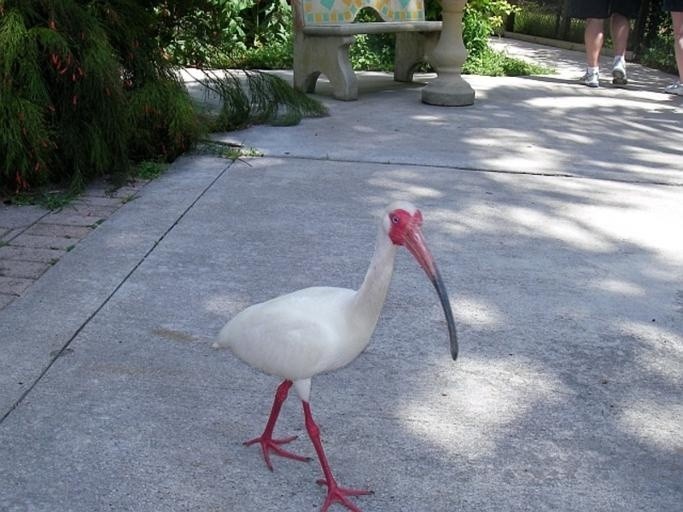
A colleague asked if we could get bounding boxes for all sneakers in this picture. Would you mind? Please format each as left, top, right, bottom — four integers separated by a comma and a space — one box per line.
663, 83, 683, 95
610, 64, 628, 85
579, 70, 600, 87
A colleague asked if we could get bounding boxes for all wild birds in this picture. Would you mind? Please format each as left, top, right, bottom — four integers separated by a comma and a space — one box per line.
208, 198, 463, 512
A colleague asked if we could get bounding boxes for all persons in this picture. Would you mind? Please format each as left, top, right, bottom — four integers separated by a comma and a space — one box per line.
570, 0, 638, 87
653, 1, 682, 96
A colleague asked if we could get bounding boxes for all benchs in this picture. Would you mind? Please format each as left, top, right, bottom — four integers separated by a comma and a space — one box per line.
290, 0, 466, 103
